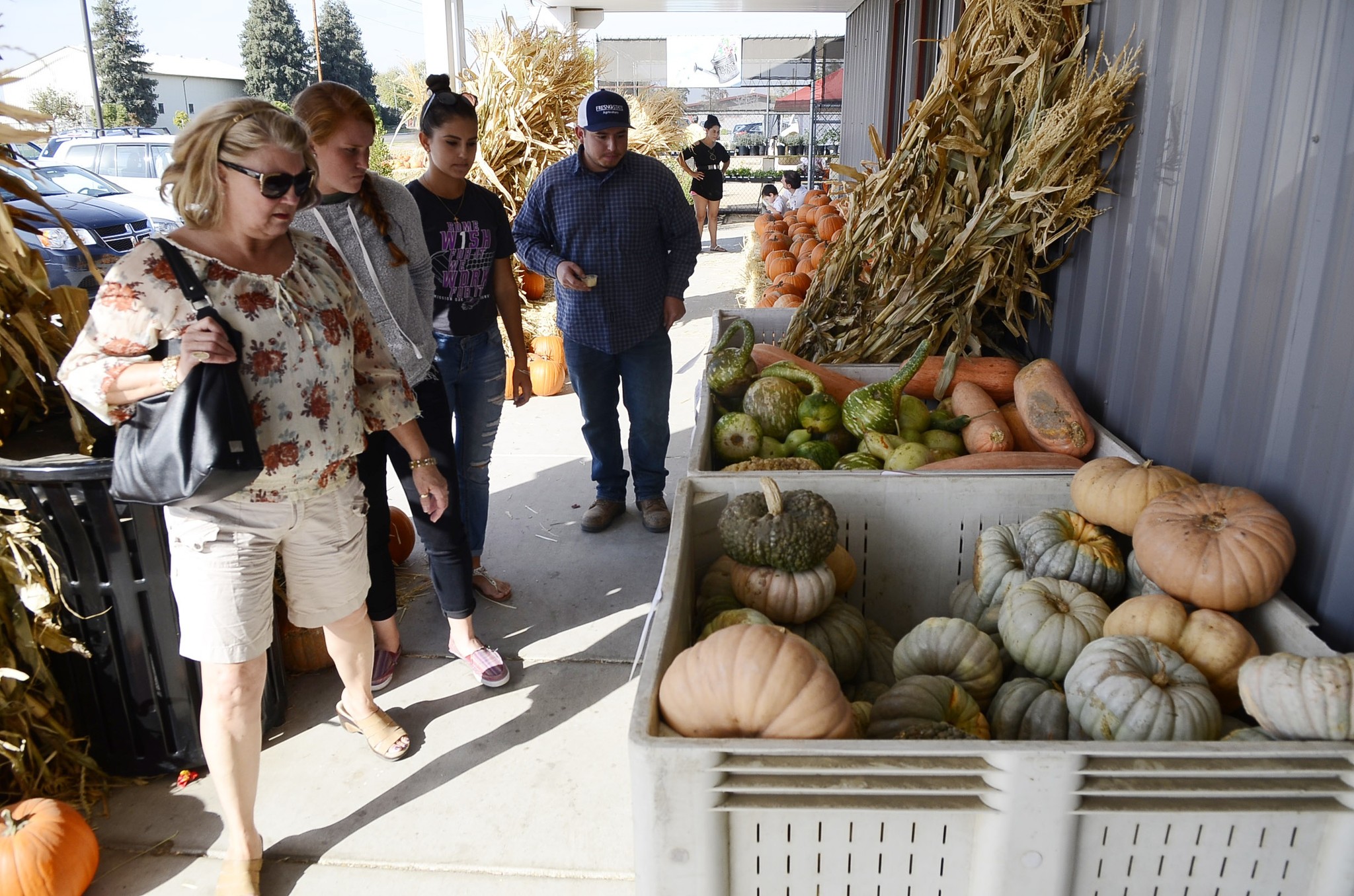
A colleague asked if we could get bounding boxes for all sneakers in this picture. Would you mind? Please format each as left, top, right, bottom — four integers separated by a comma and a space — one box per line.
636, 497, 671, 533
581, 499, 626, 532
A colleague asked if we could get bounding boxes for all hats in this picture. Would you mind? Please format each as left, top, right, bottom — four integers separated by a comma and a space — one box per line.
578, 88, 636, 132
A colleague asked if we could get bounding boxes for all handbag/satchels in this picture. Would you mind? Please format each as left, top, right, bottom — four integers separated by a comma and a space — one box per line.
108, 237, 264, 506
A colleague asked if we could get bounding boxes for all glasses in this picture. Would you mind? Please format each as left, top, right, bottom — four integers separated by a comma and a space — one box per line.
217, 157, 312, 200
421, 90, 478, 127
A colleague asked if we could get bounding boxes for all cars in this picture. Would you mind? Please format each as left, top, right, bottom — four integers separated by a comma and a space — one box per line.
731, 123, 800, 144
0, 125, 194, 313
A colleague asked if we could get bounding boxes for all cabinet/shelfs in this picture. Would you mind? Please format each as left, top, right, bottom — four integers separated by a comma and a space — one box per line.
723, 153, 840, 184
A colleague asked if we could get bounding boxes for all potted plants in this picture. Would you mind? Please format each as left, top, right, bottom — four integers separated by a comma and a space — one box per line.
720, 129, 841, 156
723, 167, 803, 183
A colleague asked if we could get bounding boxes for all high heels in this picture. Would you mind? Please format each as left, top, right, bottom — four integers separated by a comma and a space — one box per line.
336, 699, 410, 761
213, 832, 262, 896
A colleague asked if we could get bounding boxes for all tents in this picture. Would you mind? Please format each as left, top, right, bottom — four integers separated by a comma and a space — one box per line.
767, 66, 844, 148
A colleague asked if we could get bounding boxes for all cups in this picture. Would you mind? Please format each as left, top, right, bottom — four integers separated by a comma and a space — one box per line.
581, 274, 598, 287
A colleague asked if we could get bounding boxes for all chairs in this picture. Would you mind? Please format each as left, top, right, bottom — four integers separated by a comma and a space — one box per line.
120, 154, 145, 177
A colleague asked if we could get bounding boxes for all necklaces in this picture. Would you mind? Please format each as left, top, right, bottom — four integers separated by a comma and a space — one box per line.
422, 174, 466, 223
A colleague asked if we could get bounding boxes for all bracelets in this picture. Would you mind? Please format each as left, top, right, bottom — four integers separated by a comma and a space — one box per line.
516, 368, 530, 376
409, 457, 437, 469
162, 354, 182, 390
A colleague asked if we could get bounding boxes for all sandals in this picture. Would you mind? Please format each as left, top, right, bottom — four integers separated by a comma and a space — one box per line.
472, 566, 512, 602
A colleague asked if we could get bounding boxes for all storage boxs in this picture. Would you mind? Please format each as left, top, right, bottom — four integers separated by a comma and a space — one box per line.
626, 307, 1353, 895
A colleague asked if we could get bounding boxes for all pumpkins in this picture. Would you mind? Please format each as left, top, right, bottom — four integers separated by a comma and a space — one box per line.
275, 595, 335, 672
658, 454, 1354, 743
503, 334, 569, 401
0, 796, 99, 896
519, 269, 546, 300
754, 188, 853, 309
704, 319, 1093, 470
387, 506, 415, 565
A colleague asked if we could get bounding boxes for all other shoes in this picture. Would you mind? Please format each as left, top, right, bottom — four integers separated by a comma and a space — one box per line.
448, 639, 510, 687
371, 640, 402, 691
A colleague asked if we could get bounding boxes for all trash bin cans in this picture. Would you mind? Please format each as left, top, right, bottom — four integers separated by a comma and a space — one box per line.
0, 453, 287, 776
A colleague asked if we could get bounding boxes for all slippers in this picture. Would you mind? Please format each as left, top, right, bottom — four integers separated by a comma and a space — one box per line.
710, 246, 727, 252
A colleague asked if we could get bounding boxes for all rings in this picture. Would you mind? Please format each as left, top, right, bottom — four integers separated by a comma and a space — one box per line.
420, 493, 429, 498
191, 351, 210, 365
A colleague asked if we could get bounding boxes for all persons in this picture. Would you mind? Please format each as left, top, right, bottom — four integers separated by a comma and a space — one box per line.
55, 97, 449, 894
511, 88, 703, 533
780, 170, 808, 210
761, 184, 788, 215
403, 75, 534, 603
292, 80, 511, 689
676, 114, 730, 252
